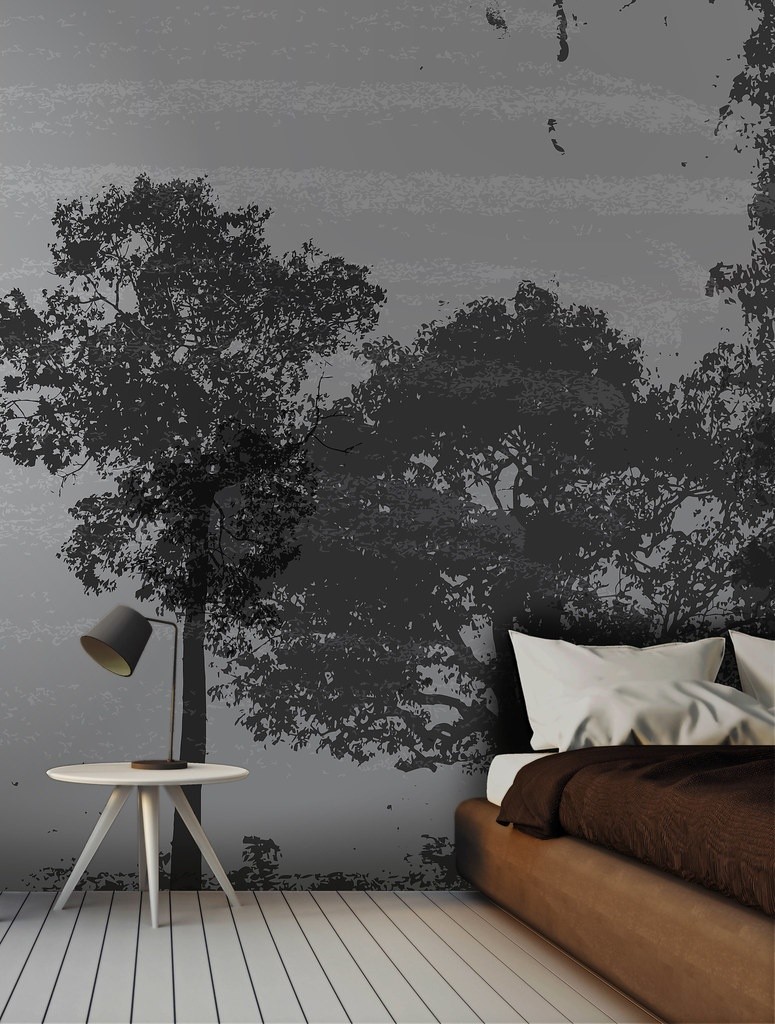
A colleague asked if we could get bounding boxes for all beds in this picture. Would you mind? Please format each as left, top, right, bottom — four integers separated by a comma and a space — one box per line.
454, 743, 775, 1024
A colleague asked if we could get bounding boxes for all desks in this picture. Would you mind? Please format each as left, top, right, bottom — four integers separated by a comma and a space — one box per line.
45, 762, 249, 928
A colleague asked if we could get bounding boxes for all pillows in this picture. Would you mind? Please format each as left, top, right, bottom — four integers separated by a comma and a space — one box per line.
558, 680, 775, 752
728, 629, 775, 719
506, 628, 726, 750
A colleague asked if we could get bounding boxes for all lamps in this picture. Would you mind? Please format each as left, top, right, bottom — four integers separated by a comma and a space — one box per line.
80, 605, 188, 770
